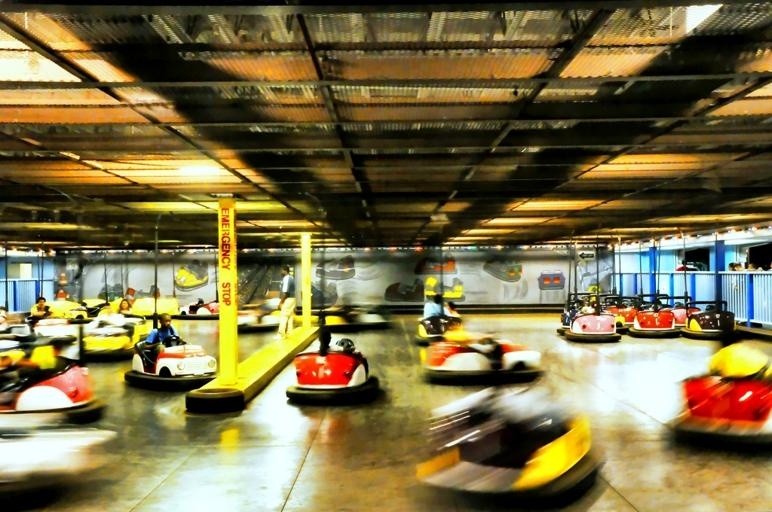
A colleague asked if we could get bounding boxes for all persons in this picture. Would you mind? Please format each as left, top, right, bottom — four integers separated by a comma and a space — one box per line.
676, 260, 688, 271
424, 294, 451, 318
319, 327, 345, 356
708, 326, 772, 383
0, 263, 296, 371
728, 260, 772, 271
447, 302, 460, 318
